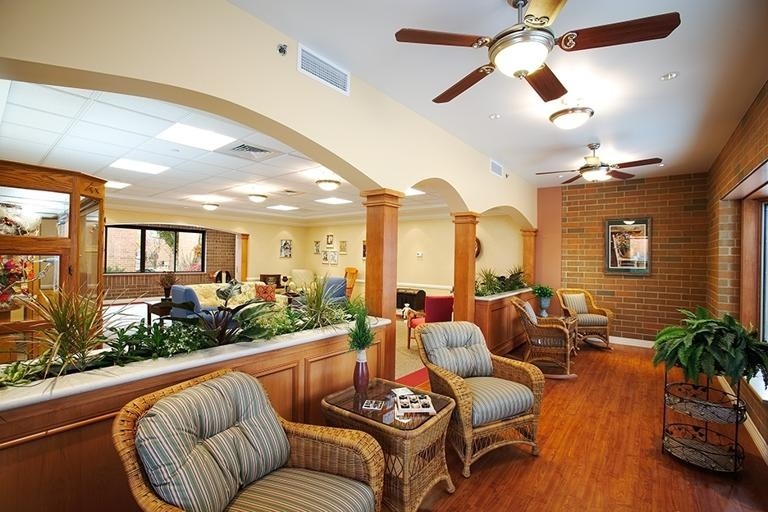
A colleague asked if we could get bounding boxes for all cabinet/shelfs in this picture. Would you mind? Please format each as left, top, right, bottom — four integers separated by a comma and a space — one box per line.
0, 159, 107, 364
661, 358, 747, 480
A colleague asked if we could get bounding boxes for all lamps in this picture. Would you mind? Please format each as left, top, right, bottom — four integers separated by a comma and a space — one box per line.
549, 106, 595, 130
316, 180, 342, 191
201, 204, 219, 210
582, 169, 606, 183
247, 193, 268, 203
494, 41, 548, 80
623, 220, 635, 225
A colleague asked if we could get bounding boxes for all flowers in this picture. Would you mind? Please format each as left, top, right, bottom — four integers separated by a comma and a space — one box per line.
159, 271, 184, 288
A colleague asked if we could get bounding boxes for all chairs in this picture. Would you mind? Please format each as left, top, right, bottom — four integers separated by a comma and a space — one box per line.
292, 277, 346, 306
555, 288, 616, 351
111, 367, 386, 512
414, 320, 545, 479
408, 295, 454, 349
510, 295, 579, 380
170, 285, 229, 321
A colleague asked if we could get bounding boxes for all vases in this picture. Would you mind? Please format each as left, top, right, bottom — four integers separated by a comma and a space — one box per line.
164, 287, 171, 298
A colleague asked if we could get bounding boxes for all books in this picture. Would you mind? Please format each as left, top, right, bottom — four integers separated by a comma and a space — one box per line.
360, 384, 438, 428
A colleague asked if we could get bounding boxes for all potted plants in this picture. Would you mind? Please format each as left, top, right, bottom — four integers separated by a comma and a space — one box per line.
346, 304, 377, 392
532, 283, 554, 318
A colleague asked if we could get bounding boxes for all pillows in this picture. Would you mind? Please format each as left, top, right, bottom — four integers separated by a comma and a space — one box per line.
134, 371, 291, 512
561, 293, 588, 314
420, 321, 494, 378
255, 284, 277, 302
522, 301, 538, 325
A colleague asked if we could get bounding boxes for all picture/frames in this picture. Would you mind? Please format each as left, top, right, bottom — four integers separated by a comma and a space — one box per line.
280, 234, 367, 265
603, 214, 652, 277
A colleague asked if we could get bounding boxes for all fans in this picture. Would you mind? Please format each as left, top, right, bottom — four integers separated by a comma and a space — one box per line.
536, 143, 663, 185
395, 0, 681, 103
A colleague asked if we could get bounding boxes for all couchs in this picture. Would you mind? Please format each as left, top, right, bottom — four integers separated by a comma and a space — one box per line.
170, 281, 289, 330
